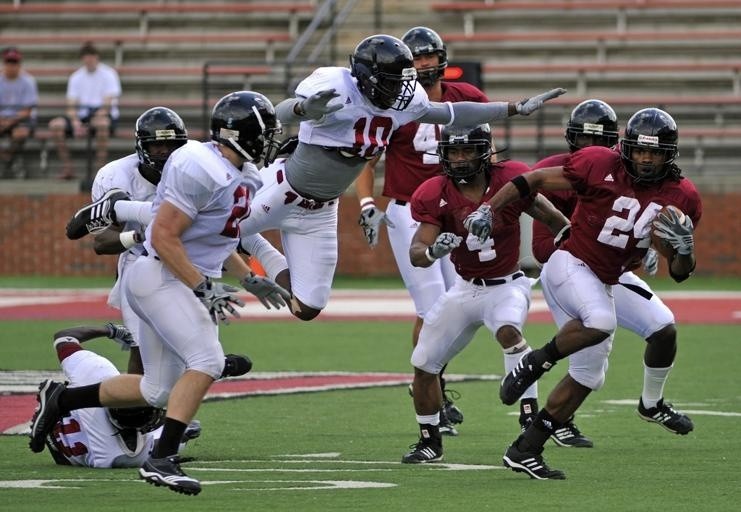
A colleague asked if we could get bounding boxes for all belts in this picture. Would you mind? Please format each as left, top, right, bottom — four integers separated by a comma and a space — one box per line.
465, 271, 529, 289
277, 162, 338, 210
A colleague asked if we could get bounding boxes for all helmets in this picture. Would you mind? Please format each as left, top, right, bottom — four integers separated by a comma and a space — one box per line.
347, 34, 417, 112
435, 123, 492, 183
563, 98, 619, 154
134, 104, 187, 171
101, 399, 166, 433
401, 27, 449, 89
209, 89, 283, 168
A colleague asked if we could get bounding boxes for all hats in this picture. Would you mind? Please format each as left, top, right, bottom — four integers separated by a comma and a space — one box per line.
1, 46, 20, 62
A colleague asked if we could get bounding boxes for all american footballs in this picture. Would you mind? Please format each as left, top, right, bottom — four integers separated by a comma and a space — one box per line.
652, 205, 686, 259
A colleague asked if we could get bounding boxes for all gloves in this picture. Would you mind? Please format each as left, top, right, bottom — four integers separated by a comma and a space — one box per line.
192, 275, 246, 326
241, 273, 292, 311
179, 420, 201, 442
105, 321, 137, 351
642, 249, 658, 276
463, 203, 495, 244
512, 85, 567, 117
358, 196, 394, 248
652, 208, 694, 255
299, 86, 344, 120
424, 232, 462, 261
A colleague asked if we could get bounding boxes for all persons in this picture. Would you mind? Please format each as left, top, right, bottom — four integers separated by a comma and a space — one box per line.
49, 47, 122, 188
0, 46, 39, 180
27, 91, 286, 499
69, 33, 567, 320
359, 27, 494, 435
402, 99, 705, 481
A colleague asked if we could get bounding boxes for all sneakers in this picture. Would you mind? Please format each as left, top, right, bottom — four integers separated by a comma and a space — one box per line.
634, 394, 694, 435
220, 352, 252, 378
497, 351, 542, 406
400, 380, 537, 464
621, 106, 683, 189
63, 187, 131, 241
137, 454, 200, 497
502, 439, 567, 483
26, 379, 71, 454
549, 418, 595, 450
